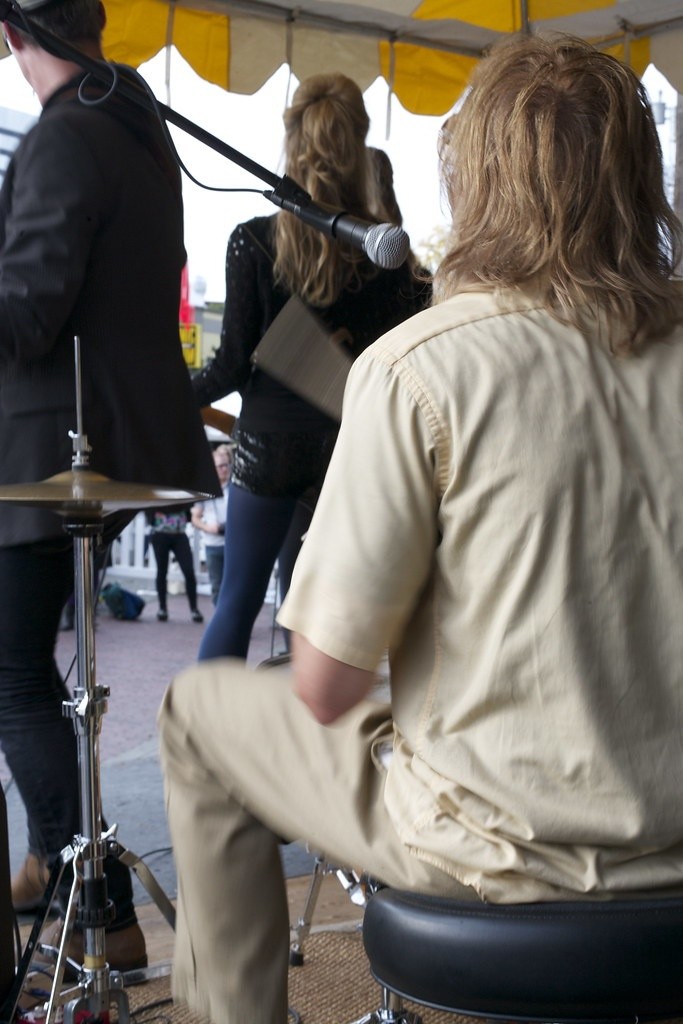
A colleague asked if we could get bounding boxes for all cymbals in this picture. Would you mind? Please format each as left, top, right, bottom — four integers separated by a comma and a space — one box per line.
0, 470, 220, 515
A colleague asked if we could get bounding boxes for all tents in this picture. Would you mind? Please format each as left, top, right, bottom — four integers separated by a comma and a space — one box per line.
0, 1, 683, 285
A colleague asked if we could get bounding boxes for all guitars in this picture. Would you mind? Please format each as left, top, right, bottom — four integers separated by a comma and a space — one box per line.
201, 402, 238, 436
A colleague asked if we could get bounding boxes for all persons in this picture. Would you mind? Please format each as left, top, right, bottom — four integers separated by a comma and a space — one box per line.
190, 73, 435, 669
156, 30, 683, 1024
59, 570, 98, 631
0, 0, 189, 986
145, 444, 233, 623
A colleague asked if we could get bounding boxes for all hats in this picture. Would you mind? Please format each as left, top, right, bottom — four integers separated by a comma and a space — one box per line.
0, 0, 47, 60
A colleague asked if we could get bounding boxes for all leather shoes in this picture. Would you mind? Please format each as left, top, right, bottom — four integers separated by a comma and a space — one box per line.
11, 853, 60, 910
14, 917, 148, 984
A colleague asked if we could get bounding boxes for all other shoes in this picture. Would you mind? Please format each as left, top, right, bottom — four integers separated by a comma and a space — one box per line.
191, 608, 202, 621
157, 611, 167, 622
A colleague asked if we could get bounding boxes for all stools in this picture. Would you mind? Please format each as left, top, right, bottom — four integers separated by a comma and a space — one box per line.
351, 883, 683, 1022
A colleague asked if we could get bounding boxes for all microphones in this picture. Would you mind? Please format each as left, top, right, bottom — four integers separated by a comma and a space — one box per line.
262, 190, 410, 268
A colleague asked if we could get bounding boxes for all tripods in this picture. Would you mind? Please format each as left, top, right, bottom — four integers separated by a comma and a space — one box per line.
0, 518, 177, 1024
